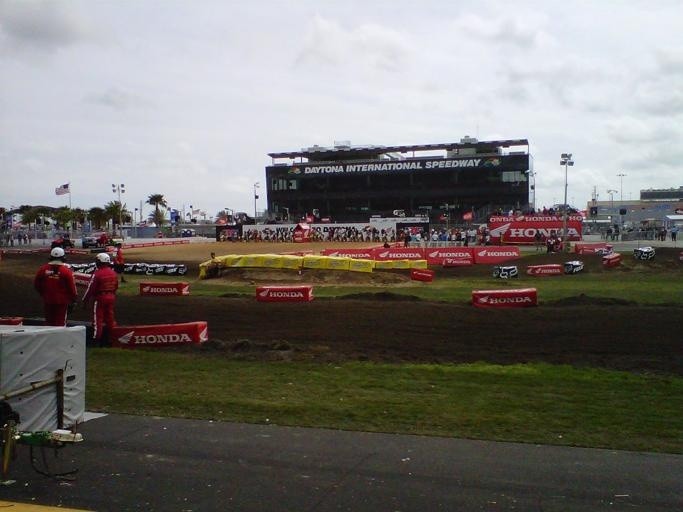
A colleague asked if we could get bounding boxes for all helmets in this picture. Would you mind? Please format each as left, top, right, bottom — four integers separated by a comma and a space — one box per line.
51, 247, 65, 257
95, 253, 110, 263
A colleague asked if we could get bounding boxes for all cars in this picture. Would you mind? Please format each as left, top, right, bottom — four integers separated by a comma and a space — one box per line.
51, 237, 74, 249
82, 232, 106, 248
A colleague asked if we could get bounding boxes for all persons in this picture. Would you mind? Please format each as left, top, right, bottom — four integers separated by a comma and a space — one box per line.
600, 221, 680, 241
5, 230, 32, 246
34, 247, 79, 327
83, 252, 119, 345
222, 204, 563, 254
113, 243, 128, 283
99, 233, 107, 246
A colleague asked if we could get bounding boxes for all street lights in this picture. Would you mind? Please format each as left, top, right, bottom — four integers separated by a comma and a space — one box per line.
525, 169, 536, 214
225, 208, 234, 224
559, 153, 574, 241
111, 183, 126, 239
606, 189, 618, 212
253, 182, 260, 225
616, 173, 626, 201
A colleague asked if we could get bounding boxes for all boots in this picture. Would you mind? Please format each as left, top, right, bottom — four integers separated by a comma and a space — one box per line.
121, 277, 128, 282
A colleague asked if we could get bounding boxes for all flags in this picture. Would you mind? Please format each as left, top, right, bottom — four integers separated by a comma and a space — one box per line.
55, 183, 70, 195
